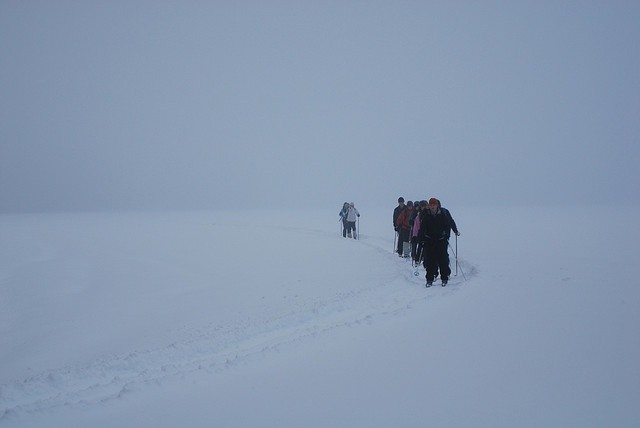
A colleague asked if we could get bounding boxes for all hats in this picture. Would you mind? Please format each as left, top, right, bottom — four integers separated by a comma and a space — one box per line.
414, 201, 419, 207
419, 200, 428, 206
429, 198, 439, 207
398, 197, 404, 203
350, 202, 354, 205
407, 200, 413, 205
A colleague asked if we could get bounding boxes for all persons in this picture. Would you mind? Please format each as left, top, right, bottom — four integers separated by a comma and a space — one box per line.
339, 202, 350, 238
345, 201, 360, 239
392, 196, 460, 288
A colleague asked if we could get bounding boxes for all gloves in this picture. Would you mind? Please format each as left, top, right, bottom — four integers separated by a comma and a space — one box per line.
357, 213, 360, 217
395, 226, 398, 231
420, 236, 429, 246
440, 233, 449, 243
455, 231, 460, 236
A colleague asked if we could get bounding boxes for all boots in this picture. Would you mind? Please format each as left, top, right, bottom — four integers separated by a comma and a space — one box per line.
353, 230, 357, 238
348, 233, 352, 238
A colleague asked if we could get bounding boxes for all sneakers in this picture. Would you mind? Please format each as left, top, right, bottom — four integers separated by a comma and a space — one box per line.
441, 277, 448, 287
398, 252, 402, 257
447, 274, 451, 279
415, 260, 420, 266
404, 254, 411, 259
426, 279, 433, 287
433, 274, 437, 282
